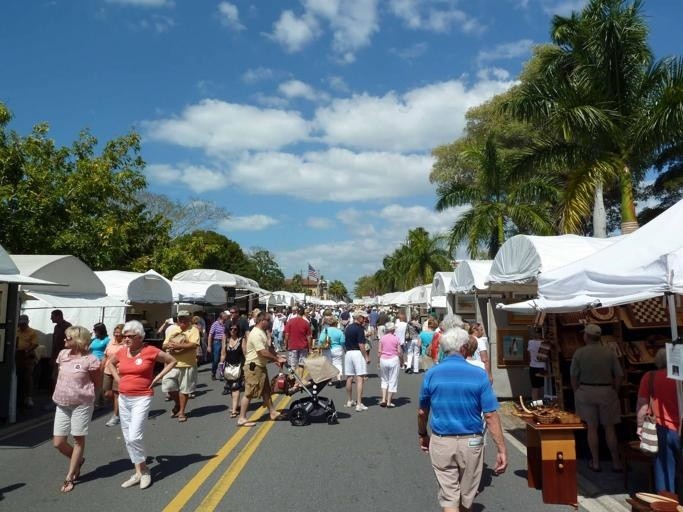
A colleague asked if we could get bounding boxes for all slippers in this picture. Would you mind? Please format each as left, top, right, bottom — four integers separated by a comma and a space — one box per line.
612, 463, 632, 473
588, 460, 601, 472
237, 419, 257, 428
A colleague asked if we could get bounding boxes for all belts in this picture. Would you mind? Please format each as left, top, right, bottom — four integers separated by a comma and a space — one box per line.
431, 429, 483, 436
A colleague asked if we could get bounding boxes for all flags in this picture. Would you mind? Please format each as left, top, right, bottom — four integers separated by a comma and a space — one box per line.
308, 264, 319, 282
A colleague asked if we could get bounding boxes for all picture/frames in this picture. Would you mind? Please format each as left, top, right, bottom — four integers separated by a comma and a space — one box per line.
496, 328, 533, 368
508, 298, 537, 325
453, 293, 477, 315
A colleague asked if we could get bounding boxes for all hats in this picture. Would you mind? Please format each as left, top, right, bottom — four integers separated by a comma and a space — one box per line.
177, 310, 190, 320
383, 321, 399, 332
19, 314, 28, 324
584, 324, 603, 336
353, 309, 369, 318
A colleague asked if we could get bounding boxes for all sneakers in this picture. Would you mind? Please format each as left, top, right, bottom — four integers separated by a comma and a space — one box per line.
345, 399, 355, 408
178, 413, 187, 423
121, 474, 142, 488
386, 402, 397, 408
355, 403, 368, 412
377, 401, 386, 406
140, 472, 154, 489
106, 415, 121, 428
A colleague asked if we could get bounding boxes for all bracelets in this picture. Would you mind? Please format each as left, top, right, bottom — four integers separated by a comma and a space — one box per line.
418, 431, 427, 436
637, 426, 643, 427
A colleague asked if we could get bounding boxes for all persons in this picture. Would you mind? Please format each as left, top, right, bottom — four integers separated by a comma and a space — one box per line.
50, 325, 101, 493
417, 326, 507, 512
570, 324, 632, 473
635, 347, 683, 495
527, 328, 546, 401
107, 319, 178, 490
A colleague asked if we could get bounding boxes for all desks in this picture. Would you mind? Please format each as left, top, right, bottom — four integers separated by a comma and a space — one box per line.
518, 414, 585, 504
625, 497, 657, 512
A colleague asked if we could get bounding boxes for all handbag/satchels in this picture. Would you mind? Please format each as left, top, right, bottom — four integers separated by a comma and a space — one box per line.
405, 323, 412, 340
321, 329, 331, 352
269, 364, 290, 396
639, 374, 659, 457
223, 361, 241, 380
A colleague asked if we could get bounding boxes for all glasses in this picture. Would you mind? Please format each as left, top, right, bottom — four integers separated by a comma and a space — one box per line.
229, 327, 236, 330
122, 334, 138, 340
263, 317, 270, 321
64, 335, 72, 340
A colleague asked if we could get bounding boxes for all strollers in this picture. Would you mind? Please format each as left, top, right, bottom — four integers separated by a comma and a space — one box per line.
275, 354, 340, 426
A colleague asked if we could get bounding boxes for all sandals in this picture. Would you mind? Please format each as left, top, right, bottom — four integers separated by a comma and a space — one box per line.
61, 476, 76, 493
230, 410, 240, 418
